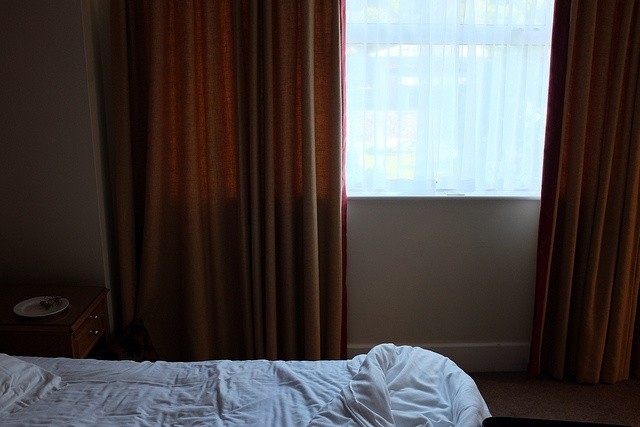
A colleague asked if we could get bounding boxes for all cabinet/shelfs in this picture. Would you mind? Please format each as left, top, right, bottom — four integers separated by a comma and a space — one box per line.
0, 284, 111, 360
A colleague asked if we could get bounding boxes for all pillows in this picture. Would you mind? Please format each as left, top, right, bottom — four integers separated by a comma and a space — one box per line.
0, 350, 62, 426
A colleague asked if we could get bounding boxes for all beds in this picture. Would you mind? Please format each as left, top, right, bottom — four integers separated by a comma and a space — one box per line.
0, 342, 492, 426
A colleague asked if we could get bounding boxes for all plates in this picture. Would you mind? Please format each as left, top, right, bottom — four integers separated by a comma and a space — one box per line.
13, 294, 70, 318
